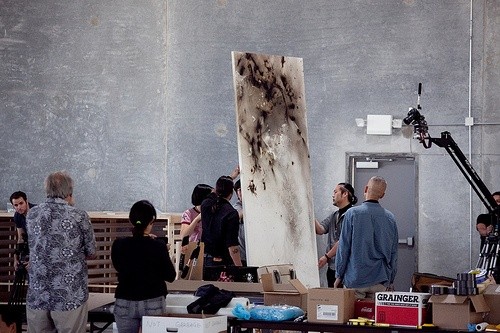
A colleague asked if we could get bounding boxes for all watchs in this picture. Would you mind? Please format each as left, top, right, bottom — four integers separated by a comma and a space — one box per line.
325, 254, 332, 263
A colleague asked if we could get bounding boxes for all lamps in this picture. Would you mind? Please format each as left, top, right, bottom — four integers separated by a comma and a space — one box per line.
355, 114, 403, 135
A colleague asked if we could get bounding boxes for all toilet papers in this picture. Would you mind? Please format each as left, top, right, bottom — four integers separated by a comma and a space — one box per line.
475, 322, 489, 332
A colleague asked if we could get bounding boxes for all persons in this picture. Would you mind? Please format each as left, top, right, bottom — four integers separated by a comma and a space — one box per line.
0, 305, 21, 333
9, 191, 37, 285
476, 191, 500, 285
26, 172, 97, 333
111, 200, 177, 333
232, 179, 247, 266
179, 183, 215, 242
314, 183, 358, 289
333, 176, 399, 300
201, 167, 243, 281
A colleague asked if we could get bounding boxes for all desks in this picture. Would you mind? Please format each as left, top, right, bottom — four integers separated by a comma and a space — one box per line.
0, 292, 116, 333
167, 313, 500, 333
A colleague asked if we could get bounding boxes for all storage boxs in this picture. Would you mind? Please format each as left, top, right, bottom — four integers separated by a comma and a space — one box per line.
202, 264, 500, 331
141, 315, 228, 333
